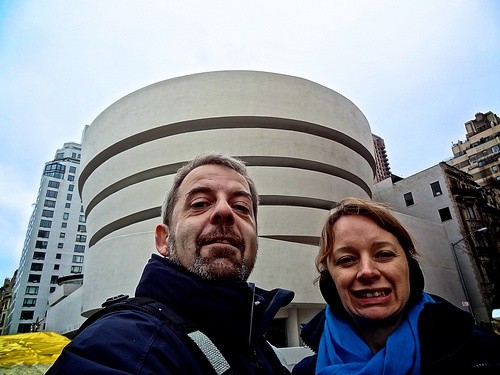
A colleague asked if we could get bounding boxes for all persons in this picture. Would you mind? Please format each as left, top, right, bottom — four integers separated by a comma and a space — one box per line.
44, 151, 295, 375
292, 197, 500, 375
